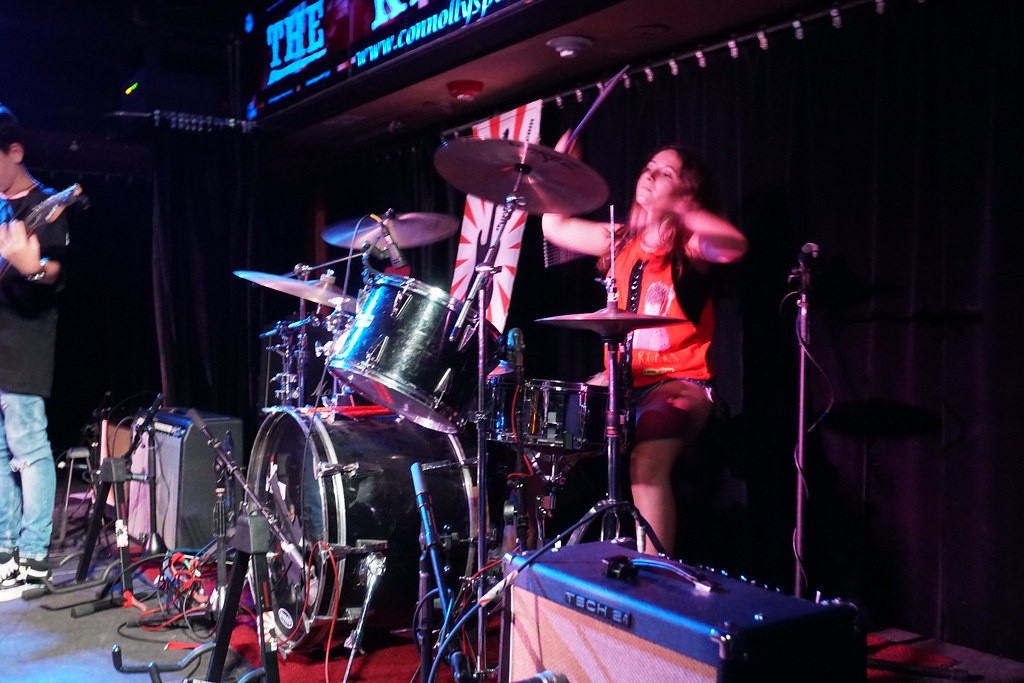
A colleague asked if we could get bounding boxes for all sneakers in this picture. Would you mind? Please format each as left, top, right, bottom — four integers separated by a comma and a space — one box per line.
0, 555, 53, 602
0, 545, 20, 589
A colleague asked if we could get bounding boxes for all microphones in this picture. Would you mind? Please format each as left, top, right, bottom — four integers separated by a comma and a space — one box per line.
512, 669, 569, 683
282, 544, 316, 583
506, 327, 525, 386
797, 241, 819, 294
381, 224, 408, 269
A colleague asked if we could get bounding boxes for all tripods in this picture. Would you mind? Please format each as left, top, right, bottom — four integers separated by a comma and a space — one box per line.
53, 391, 172, 562
563, 339, 666, 558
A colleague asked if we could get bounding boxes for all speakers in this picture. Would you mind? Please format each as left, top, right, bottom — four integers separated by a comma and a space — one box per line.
98, 409, 245, 557
497, 540, 870, 683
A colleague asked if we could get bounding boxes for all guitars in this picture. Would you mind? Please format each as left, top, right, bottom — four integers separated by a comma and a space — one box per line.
0, 184, 84, 279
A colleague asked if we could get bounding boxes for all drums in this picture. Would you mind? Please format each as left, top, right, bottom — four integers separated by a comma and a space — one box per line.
326, 275, 504, 434
483, 375, 637, 453
245, 409, 476, 659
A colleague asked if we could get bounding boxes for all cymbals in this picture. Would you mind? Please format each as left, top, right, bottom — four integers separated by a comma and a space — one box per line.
432, 137, 609, 216
533, 306, 692, 337
320, 210, 461, 250
232, 269, 357, 315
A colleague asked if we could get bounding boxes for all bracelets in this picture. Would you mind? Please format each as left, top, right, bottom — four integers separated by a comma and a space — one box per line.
24, 255, 47, 282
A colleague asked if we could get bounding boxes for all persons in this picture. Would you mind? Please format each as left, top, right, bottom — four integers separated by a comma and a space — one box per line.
541, 130, 746, 560
0, 105, 69, 602
